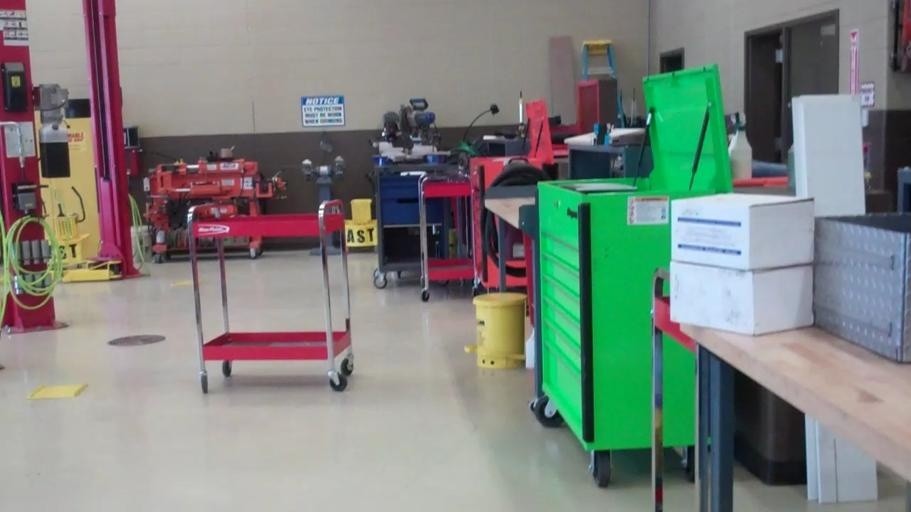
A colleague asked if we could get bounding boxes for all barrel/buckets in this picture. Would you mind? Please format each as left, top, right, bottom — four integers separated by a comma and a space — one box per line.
472, 292, 528, 369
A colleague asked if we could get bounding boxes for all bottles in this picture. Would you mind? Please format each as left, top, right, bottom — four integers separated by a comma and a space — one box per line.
728, 125, 752, 178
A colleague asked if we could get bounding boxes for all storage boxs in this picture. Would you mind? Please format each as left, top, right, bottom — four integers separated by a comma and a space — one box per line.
669, 261, 815, 337
671, 191, 813, 274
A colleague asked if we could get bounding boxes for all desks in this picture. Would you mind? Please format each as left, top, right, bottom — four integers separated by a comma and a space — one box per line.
679, 324, 911, 511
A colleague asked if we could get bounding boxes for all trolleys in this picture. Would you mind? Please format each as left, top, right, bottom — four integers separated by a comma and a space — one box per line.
368, 150, 452, 289
418, 166, 484, 302
187, 197, 356, 396
